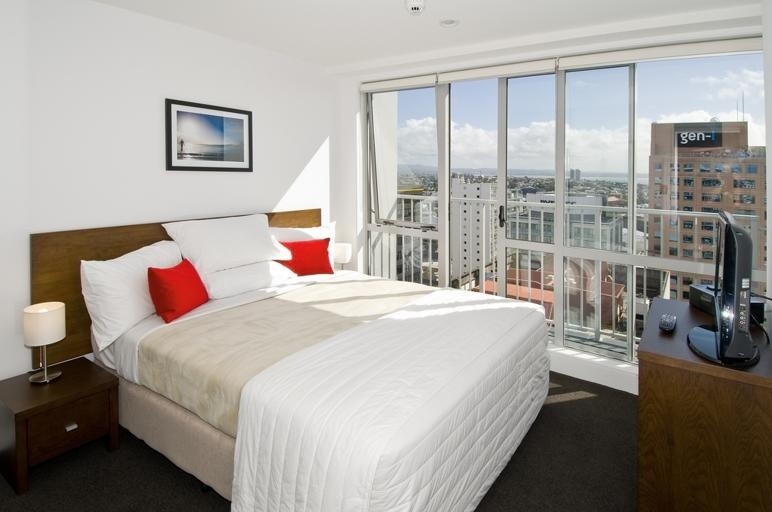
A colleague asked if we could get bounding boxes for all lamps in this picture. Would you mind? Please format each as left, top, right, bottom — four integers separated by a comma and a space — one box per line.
23, 300, 68, 385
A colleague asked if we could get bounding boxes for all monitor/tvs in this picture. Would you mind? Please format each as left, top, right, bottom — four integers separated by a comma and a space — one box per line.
687, 209, 760, 370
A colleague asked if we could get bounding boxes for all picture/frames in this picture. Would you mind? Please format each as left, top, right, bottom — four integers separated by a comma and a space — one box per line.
163, 97, 254, 174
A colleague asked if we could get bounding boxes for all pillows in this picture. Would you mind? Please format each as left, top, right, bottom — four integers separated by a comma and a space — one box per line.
78, 239, 184, 360
272, 237, 335, 276
269, 219, 338, 271
160, 212, 293, 276
146, 257, 211, 324
200, 260, 299, 301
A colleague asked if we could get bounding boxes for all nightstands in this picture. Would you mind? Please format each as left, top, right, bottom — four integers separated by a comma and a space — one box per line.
1, 356, 121, 497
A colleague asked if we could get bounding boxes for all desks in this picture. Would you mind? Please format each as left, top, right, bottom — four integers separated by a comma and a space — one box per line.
635, 295, 770, 510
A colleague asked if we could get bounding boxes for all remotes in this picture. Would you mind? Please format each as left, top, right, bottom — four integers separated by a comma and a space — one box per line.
659, 312, 677, 330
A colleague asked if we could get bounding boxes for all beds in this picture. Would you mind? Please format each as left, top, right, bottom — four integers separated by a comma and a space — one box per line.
28, 208, 553, 512
